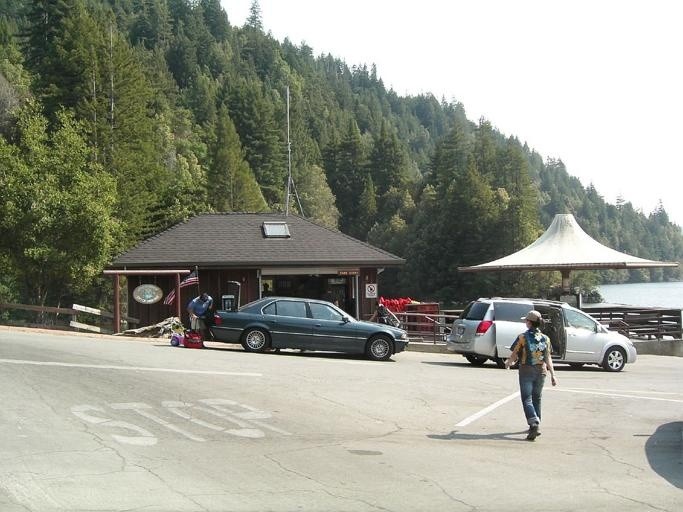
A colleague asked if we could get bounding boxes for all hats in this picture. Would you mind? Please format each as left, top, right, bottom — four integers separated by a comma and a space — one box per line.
518, 309, 542, 322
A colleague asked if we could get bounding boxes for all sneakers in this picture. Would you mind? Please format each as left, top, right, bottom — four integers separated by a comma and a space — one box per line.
525, 422, 541, 440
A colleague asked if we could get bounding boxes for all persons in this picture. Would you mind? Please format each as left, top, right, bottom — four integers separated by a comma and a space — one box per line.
262, 283, 273, 298
505, 310, 557, 439
187, 292, 213, 347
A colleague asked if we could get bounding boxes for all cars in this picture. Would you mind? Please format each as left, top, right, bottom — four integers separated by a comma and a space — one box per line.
208, 296, 409, 361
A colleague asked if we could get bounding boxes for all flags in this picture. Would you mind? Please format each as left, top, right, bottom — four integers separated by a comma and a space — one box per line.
163, 270, 199, 305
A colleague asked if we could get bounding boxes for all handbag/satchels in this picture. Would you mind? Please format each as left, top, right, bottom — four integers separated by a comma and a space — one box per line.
183, 329, 204, 347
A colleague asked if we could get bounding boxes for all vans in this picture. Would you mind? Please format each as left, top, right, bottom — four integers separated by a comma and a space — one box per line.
445, 296, 636, 372
